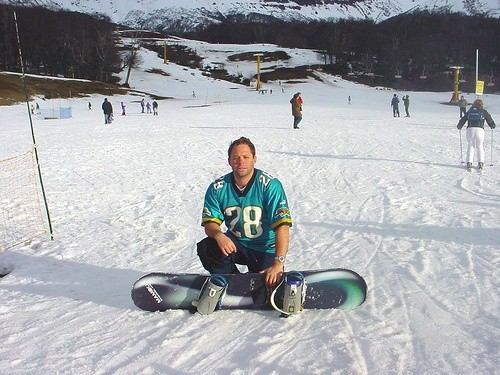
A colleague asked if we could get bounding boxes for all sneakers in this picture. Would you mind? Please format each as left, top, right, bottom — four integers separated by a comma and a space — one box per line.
466, 162, 473, 169
479, 163, 483, 170
294, 126, 299, 128
293, 124, 297, 128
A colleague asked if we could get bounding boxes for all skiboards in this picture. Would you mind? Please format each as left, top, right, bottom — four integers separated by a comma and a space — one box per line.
465, 161, 484, 173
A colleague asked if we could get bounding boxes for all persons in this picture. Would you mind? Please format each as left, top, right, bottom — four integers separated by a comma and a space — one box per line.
457, 99, 496, 170
390, 94, 400, 117
152, 101, 160, 115
458, 96, 467, 118
197, 137, 292, 288
290, 92, 304, 129
146, 102, 152, 113
121, 102, 126, 115
141, 99, 145, 113
402, 95, 410, 117
102, 98, 113, 124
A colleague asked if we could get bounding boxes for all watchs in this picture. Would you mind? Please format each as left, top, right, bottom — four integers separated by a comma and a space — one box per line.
274, 256, 286, 263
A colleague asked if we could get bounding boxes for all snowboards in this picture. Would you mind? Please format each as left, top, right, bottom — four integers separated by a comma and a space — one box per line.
130, 268, 368, 316
